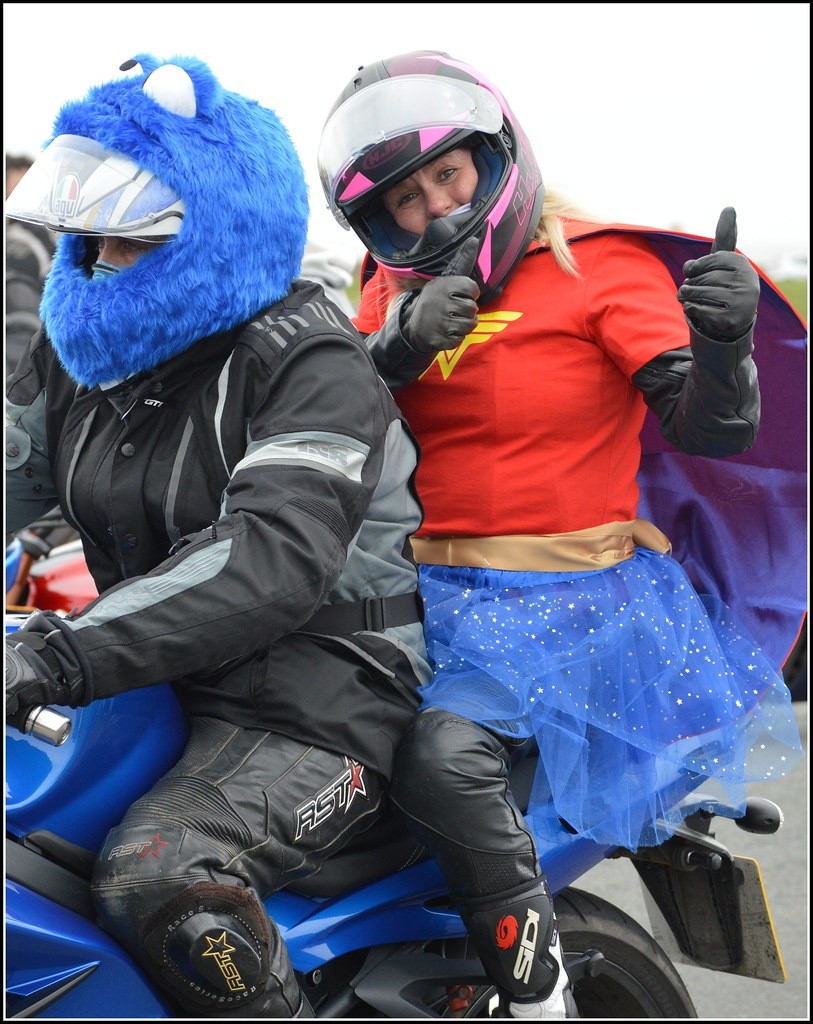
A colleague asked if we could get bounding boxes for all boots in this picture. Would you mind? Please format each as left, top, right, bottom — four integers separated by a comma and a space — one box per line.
455, 871, 579, 1018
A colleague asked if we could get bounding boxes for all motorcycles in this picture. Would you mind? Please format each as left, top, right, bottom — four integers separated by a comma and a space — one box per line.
7, 515, 783, 1020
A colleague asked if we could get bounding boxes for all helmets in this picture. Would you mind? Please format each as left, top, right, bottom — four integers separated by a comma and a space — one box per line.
315, 50, 544, 311
1, 135, 189, 394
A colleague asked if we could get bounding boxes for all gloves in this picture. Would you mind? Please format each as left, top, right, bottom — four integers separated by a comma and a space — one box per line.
677, 206, 760, 344
397, 234, 479, 353
6, 608, 94, 721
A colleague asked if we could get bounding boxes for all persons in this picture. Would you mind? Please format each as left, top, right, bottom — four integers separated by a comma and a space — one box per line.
6, 52, 431, 1019
315, 47, 763, 1019
